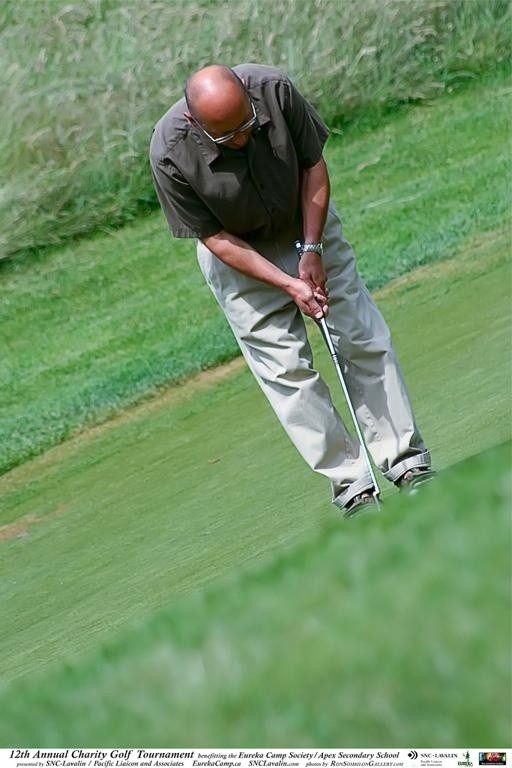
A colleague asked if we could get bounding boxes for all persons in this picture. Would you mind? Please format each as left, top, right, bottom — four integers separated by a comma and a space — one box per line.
148, 62, 437, 519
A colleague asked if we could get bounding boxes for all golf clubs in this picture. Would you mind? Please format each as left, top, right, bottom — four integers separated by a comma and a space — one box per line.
292, 240, 382, 507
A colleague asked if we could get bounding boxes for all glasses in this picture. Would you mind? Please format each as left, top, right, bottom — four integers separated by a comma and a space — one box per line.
187, 102, 257, 144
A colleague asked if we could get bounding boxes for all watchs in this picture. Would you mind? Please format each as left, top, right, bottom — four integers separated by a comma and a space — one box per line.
302, 242, 324, 256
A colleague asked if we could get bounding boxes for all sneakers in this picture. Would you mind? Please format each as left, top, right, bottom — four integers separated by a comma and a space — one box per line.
340, 493, 382, 520
396, 468, 438, 491
